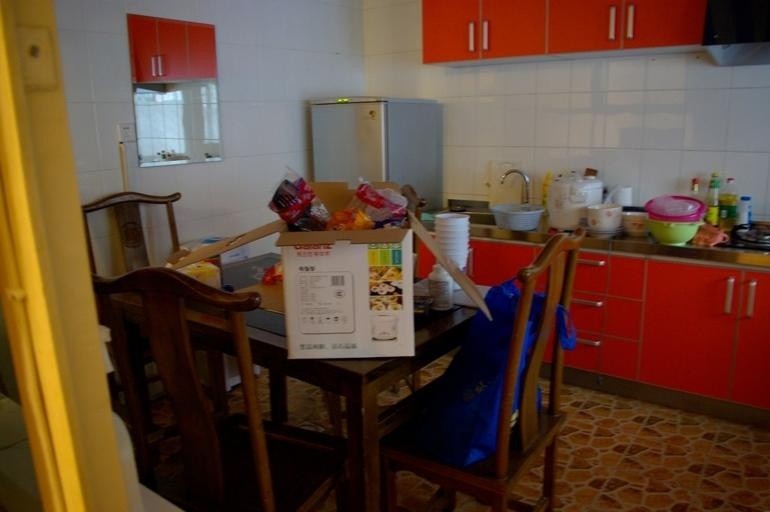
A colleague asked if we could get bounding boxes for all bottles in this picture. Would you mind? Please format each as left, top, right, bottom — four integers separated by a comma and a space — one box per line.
603, 186, 610, 204
735, 196, 752, 232
427, 265, 453, 310
718, 178, 737, 233
704, 172, 719, 227
691, 177, 699, 196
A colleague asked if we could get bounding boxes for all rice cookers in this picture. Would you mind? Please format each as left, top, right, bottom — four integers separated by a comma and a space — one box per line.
546, 166, 604, 232
371, 313, 398, 341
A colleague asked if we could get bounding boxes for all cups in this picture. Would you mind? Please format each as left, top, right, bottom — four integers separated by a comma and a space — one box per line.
434, 213, 471, 290
615, 186, 632, 207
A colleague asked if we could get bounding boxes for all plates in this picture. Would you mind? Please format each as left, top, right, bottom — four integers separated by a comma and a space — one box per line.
585, 227, 626, 239
371, 284, 395, 295
390, 281, 403, 289
371, 297, 402, 312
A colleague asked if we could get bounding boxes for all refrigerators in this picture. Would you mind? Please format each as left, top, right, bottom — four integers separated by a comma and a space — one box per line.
309, 96, 445, 211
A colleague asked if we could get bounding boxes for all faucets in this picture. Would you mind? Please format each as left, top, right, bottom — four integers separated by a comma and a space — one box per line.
501, 169, 530, 204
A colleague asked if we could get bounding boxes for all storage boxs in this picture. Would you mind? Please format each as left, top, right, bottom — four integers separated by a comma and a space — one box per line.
167, 173, 495, 361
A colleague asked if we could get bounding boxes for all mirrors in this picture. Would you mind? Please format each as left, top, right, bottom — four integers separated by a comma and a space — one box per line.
126, 13, 226, 169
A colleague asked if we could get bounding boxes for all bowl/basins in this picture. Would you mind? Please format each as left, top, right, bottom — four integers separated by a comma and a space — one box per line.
622, 212, 650, 237
648, 219, 705, 246
489, 203, 547, 233
587, 205, 622, 228
644, 195, 708, 221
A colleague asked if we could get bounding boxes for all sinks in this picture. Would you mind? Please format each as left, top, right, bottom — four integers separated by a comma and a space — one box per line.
458, 212, 497, 226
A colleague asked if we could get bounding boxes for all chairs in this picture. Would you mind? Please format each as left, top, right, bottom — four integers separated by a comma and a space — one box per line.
75, 189, 184, 273
379, 223, 590, 511
89, 267, 349, 512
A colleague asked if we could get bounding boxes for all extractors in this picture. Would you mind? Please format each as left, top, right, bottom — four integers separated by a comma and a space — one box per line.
702, 0, 770, 67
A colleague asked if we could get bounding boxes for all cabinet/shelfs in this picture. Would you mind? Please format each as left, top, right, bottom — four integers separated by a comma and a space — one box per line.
546, 2, 705, 54
419, 0, 547, 65
127, 13, 217, 83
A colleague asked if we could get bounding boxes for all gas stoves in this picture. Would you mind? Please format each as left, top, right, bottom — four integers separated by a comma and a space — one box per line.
713, 220, 770, 254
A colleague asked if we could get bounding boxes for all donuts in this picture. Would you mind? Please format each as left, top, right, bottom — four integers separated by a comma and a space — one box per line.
369, 266, 401, 311
